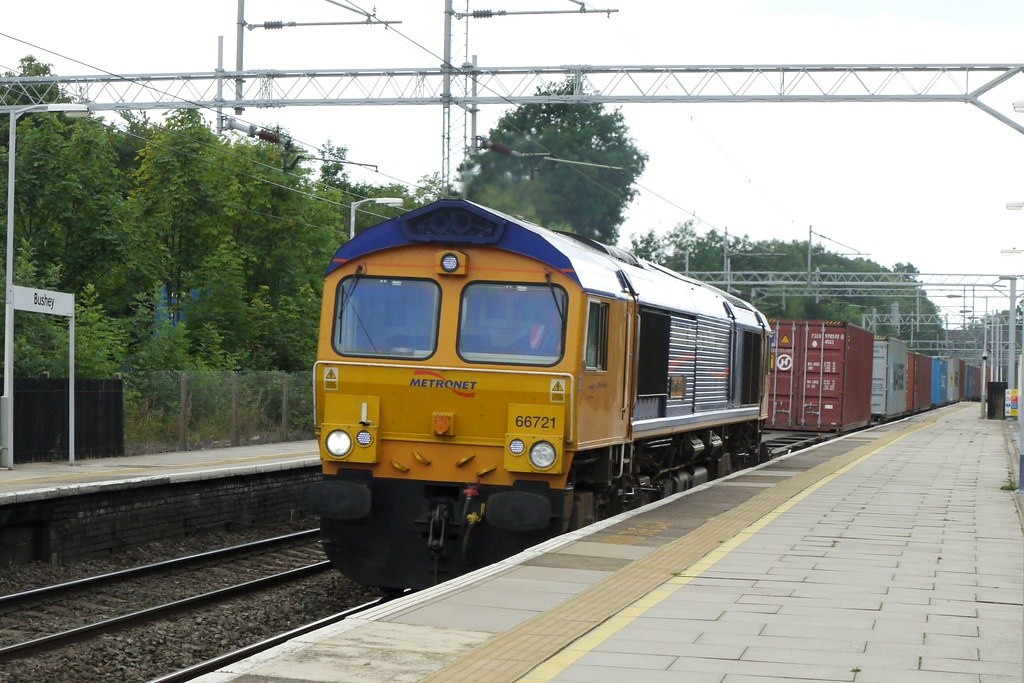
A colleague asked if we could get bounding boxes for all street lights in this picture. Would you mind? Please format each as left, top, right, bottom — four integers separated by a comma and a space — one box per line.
959, 309, 1003, 383
0, 102, 92, 470
947, 294, 989, 419
349, 197, 402, 241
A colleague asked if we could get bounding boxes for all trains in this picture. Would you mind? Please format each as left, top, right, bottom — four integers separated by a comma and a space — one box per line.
306, 198, 989, 602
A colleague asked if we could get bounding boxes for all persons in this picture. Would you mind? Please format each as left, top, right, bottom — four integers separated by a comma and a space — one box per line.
508, 305, 562, 358
